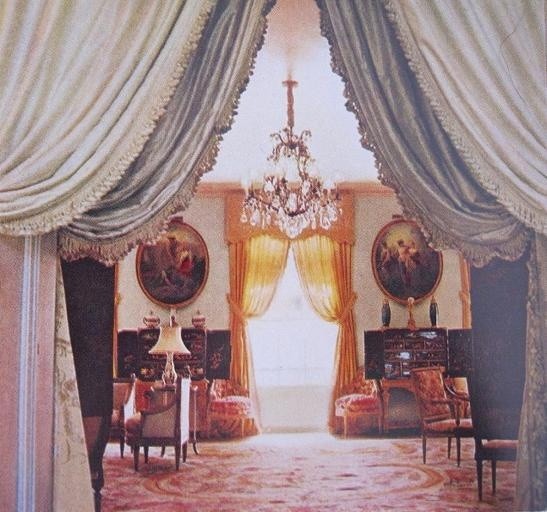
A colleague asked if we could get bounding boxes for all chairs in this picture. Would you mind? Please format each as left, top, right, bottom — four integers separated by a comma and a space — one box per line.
332, 377, 383, 441
102, 372, 256, 472
409, 365, 475, 469
460, 362, 523, 503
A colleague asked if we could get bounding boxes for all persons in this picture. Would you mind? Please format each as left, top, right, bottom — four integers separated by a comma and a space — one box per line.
376, 236, 435, 286
142, 235, 200, 300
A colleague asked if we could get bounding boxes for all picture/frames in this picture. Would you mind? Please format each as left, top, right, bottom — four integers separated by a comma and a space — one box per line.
134, 217, 212, 311
369, 214, 446, 307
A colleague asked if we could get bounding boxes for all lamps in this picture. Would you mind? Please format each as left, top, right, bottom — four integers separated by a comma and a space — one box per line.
145, 323, 192, 385
236, 69, 346, 240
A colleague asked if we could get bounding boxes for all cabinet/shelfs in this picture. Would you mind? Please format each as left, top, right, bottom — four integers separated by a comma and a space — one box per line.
446, 325, 475, 381
363, 328, 448, 384
117, 327, 231, 383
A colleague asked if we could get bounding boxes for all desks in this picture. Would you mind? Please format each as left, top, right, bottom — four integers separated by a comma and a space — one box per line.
375, 378, 454, 436
